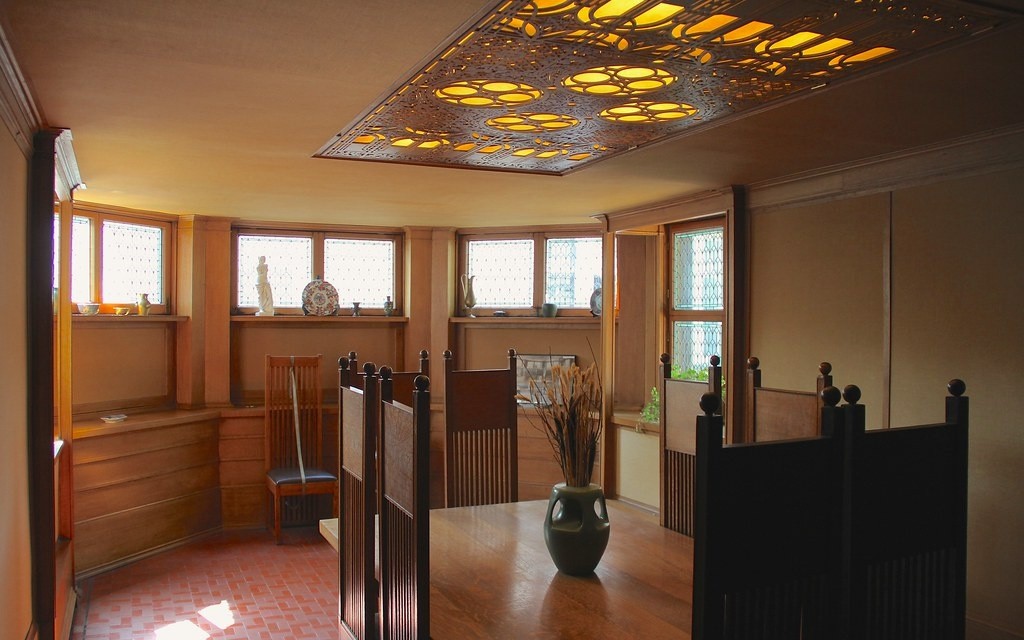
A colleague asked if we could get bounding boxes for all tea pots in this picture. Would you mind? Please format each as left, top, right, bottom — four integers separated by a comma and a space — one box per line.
461, 273, 478, 319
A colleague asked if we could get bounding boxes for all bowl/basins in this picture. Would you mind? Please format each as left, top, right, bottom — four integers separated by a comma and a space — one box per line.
114, 307, 130, 316
77, 303, 101, 316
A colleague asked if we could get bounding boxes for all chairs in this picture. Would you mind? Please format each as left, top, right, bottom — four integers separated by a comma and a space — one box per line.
262, 354, 340, 545
336, 346, 972, 640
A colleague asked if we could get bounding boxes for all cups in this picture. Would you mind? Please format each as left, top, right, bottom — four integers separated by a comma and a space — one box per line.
542, 303, 557, 317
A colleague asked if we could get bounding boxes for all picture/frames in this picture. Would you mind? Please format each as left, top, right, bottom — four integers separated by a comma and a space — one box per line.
515, 354, 576, 406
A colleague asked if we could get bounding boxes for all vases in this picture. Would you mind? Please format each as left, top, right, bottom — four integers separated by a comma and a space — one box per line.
543, 482, 612, 576
135, 293, 151, 316
384, 296, 393, 317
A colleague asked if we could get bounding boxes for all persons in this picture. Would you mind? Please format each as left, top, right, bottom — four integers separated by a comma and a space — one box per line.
255, 256, 274, 313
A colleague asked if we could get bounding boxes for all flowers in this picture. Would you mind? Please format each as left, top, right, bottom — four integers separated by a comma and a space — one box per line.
509, 331, 603, 484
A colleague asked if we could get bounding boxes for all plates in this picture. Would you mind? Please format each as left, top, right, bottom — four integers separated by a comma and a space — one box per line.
589, 288, 602, 316
301, 281, 339, 316
100, 413, 128, 423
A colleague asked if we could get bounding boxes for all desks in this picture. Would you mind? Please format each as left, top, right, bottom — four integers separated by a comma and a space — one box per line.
319, 495, 694, 640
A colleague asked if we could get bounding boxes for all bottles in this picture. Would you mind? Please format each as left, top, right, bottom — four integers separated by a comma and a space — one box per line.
383, 296, 393, 316
137, 293, 151, 315
351, 302, 362, 317
